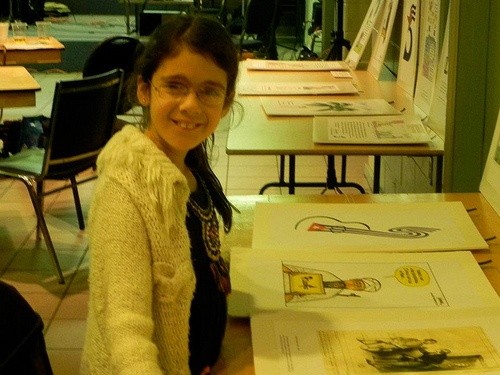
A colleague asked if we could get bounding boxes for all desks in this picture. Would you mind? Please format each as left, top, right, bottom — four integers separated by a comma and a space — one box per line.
208, 193, 500, 375
0, 36, 65, 66
226, 58, 444, 195
0, 66, 42, 109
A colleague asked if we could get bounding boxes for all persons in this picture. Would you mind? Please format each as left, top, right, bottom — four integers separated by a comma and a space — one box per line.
79, 16, 241, 375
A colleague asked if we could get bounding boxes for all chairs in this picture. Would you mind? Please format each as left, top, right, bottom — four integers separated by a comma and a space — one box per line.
0, 35, 144, 285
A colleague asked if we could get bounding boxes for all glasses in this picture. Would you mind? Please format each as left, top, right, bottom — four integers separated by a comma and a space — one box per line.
147, 79, 227, 107
